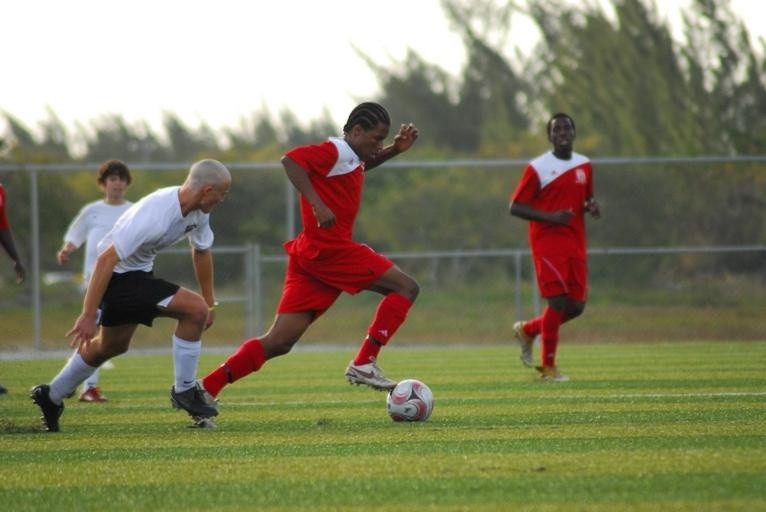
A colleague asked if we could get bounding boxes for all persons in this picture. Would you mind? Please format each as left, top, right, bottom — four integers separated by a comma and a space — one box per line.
169, 101, 421, 426
507, 111, 603, 385
1, 181, 24, 396
55, 159, 135, 407
28, 157, 235, 433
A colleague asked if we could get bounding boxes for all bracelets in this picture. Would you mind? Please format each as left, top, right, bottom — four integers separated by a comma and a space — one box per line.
207, 301, 218, 314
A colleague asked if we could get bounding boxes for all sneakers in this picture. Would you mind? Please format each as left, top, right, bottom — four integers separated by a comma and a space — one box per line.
534, 366, 571, 381
345, 360, 397, 392
29, 383, 65, 432
78, 386, 107, 401
170, 380, 219, 428
512, 320, 533, 368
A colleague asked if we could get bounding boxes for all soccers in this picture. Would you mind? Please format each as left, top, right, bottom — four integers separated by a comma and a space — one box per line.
387, 379, 434, 421
